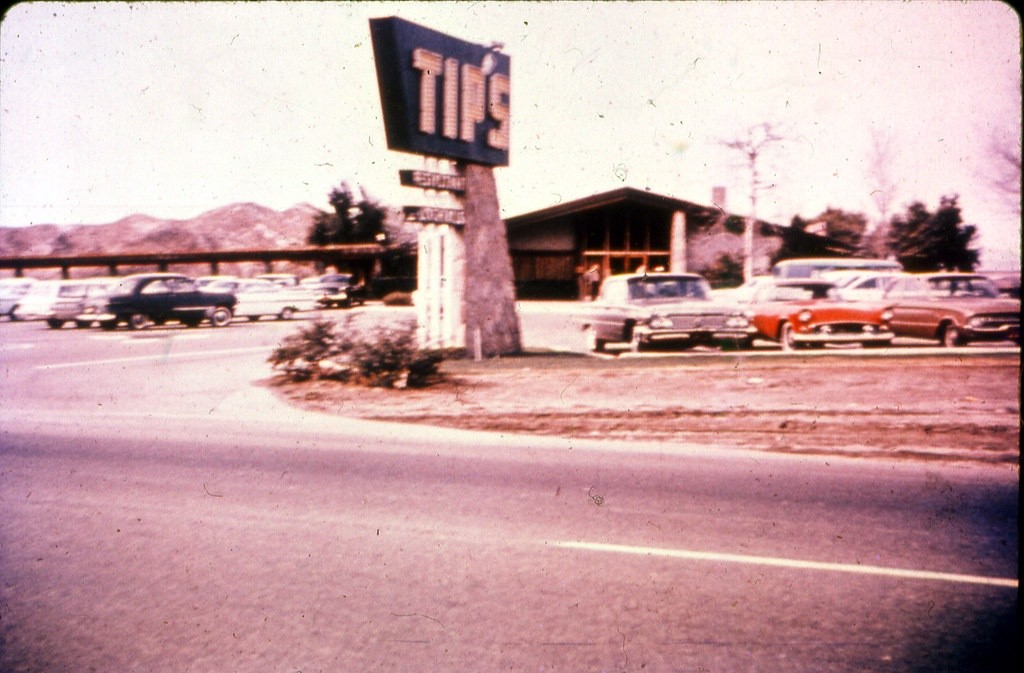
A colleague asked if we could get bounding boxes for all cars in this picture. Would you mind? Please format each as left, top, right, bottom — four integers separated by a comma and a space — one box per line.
722, 273, 898, 353
576, 270, 760, 354
0, 270, 364, 326
882, 274, 1022, 348
74, 273, 240, 328
837, 271, 916, 306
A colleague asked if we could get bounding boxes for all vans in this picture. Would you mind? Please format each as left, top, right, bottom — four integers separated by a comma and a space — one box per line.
773, 256, 903, 296
